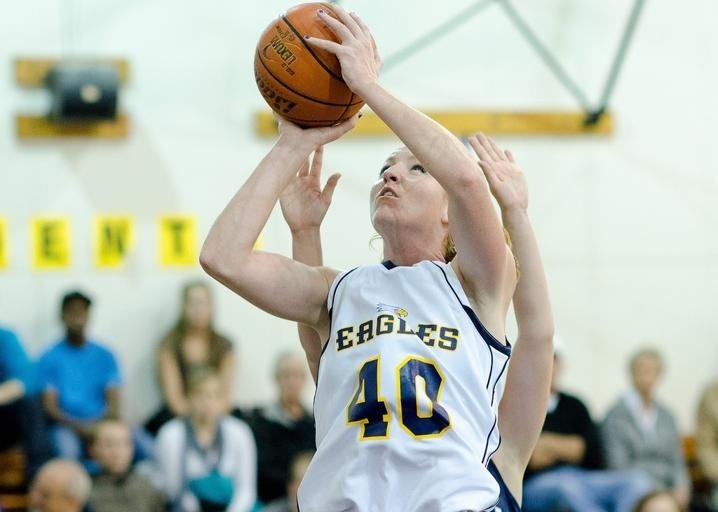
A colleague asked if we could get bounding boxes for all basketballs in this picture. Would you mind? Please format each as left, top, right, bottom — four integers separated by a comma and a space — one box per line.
254, 1, 366, 127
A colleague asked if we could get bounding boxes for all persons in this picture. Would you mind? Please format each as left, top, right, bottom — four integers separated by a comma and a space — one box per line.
197, 3, 517, 511
0, 278, 318, 512
697, 382, 718, 511
280, 130, 554, 511
525, 353, 681, 511
600, 349, 693, 510
